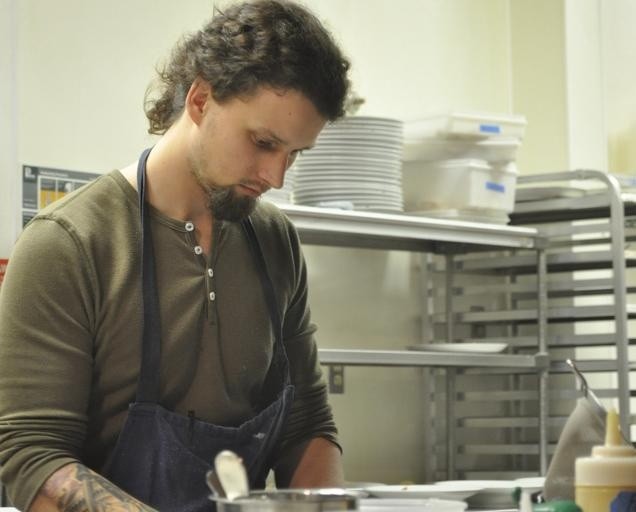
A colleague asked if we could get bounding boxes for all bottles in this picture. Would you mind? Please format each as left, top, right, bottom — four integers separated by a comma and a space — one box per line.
572, 409, 635, 512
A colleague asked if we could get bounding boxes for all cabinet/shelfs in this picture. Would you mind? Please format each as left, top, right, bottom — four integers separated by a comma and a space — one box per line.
274, 205, 551, 510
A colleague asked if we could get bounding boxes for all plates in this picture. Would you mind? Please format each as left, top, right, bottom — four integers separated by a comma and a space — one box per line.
353, 477, 547, 512
401, 343, 510, 354
258, 114, 406, 214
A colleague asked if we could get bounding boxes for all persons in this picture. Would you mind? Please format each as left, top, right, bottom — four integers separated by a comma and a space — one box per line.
1, 0, 363, 512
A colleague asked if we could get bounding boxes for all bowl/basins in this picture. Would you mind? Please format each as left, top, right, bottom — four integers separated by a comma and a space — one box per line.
207, 489, 355, 512
